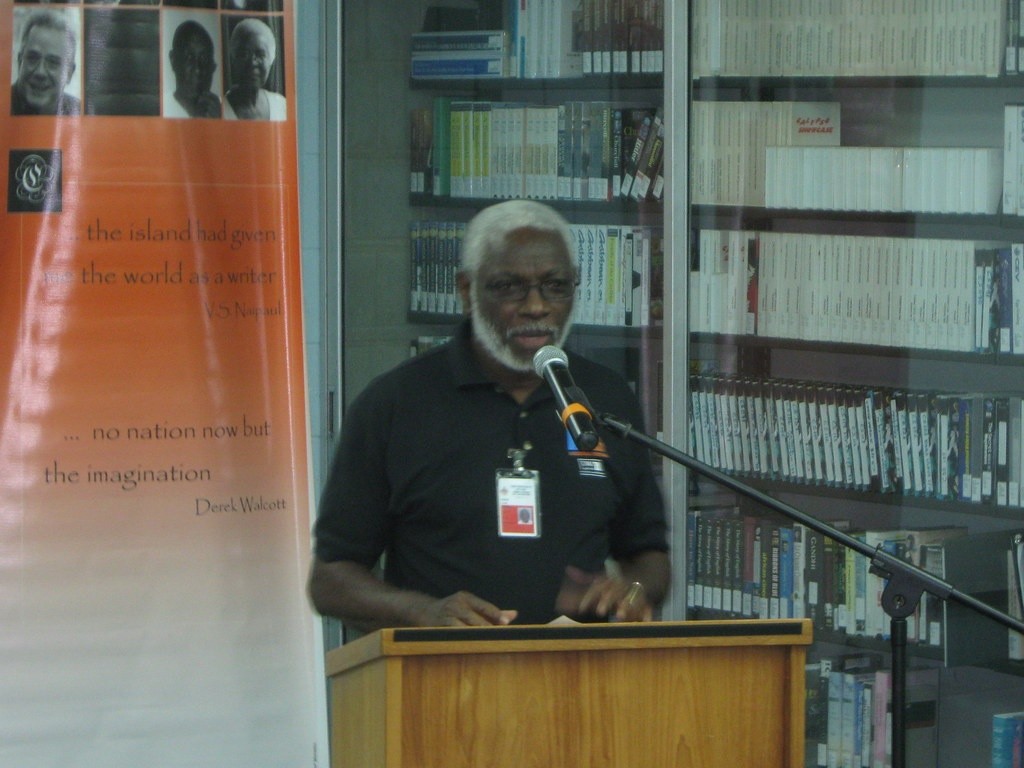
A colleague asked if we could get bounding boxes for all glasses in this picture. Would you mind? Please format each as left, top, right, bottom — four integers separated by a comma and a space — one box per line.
487, 278, 576, 302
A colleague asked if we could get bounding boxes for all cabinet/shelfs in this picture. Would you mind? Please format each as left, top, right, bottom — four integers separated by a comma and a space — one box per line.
315, 1, 1023, 680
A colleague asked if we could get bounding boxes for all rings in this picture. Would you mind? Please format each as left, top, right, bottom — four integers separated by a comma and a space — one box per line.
632, 581, 644, 588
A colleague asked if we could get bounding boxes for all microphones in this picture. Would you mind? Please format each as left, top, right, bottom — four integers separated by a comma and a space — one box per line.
534, 345, 599, 452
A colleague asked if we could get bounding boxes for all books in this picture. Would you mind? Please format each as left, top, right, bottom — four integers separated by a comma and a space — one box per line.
399, 0, 1024, 767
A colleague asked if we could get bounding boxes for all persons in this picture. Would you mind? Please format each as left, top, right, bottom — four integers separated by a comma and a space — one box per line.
225, 18, 286, 123
518, 509, 531, 524
9, 12, 81, 116
160, 18, 223, 120
305, 199, 672, 626
